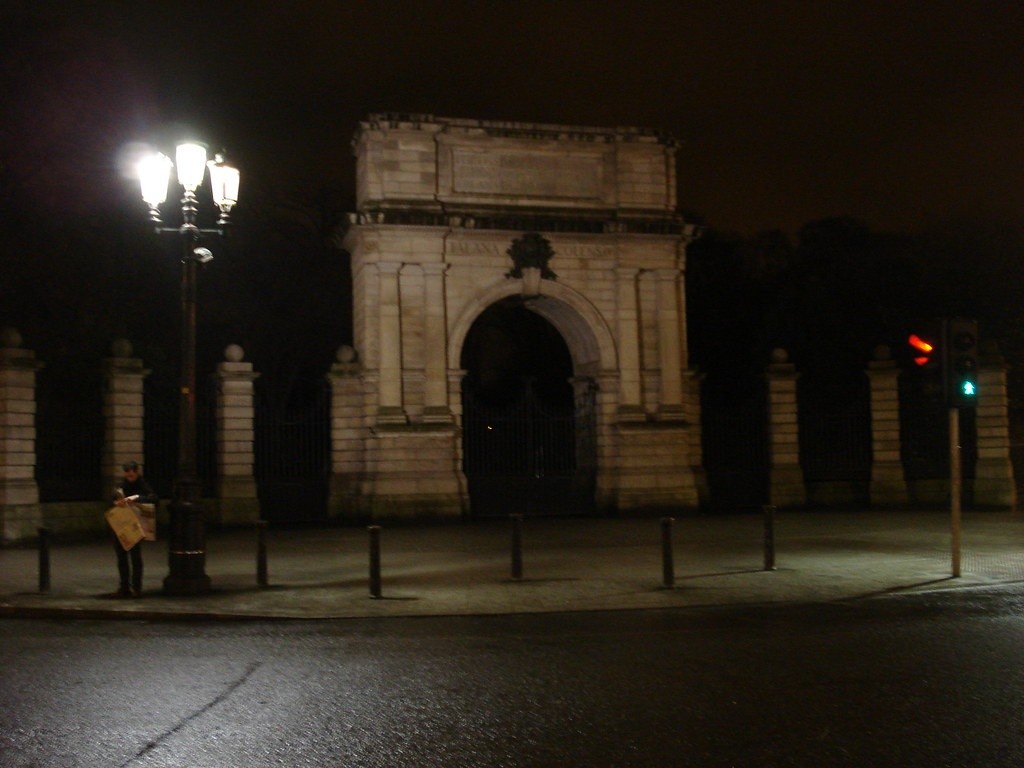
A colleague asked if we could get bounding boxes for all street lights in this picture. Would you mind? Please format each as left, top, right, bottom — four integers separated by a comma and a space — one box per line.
135, 134, 243, 600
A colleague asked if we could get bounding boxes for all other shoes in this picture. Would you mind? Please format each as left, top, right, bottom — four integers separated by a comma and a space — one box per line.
111, 587, 131, 598
134, 590, 144, 603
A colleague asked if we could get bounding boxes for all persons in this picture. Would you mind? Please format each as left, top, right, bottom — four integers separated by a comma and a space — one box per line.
108, 460, 161, 599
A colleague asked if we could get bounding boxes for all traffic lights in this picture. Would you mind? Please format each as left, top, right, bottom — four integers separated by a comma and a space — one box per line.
953, 366, 978, 404
909, 331, 941, 383
